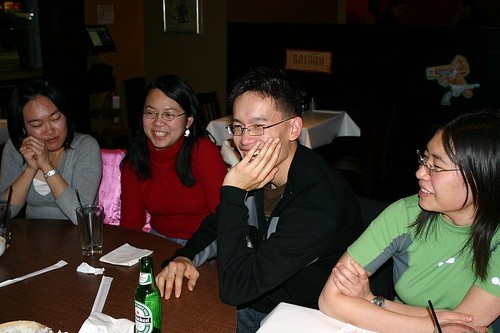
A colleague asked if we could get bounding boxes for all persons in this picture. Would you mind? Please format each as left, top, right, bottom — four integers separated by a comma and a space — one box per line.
155, 72, 363, 333
0, 82, 103, 225
317, 116, 500, 333
120, 80, 227, 268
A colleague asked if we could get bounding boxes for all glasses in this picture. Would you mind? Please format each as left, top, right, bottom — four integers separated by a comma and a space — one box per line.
225, 117, 295, 136
415, 150, 459, 177
142, 111, 187, 121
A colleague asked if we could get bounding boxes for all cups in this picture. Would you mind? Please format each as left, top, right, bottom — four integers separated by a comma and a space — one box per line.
0, 236, 6, 256
75, 205, 104, 256
0, 200, 12, 248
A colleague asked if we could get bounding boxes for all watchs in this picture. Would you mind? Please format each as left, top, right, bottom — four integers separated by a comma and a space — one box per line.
44, 169, 60, 180
370, 296, 385, 308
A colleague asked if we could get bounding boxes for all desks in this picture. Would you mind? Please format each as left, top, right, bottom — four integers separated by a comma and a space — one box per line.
206, 110, 361, 149
0, 219, 237, 333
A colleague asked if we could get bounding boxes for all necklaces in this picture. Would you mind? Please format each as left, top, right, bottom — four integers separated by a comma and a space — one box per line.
51, 149, 60, 163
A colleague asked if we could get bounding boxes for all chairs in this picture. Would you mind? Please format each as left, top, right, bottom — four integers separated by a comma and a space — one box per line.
194, 92, 222, 125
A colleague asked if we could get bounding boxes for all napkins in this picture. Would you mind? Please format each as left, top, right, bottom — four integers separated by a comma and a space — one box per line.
99, 244, 154, 267
76, 311, 135, 333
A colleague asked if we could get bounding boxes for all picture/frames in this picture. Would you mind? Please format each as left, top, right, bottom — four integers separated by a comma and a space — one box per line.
163, 0, 201, 34
97, 5, 114, 24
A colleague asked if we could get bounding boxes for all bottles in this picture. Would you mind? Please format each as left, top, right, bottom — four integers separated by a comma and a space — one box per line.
133, 256, 162, 333
